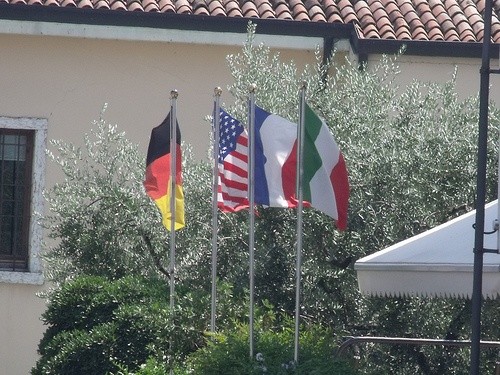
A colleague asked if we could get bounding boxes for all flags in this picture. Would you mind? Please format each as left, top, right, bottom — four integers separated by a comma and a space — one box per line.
143, 107, 186, 232
303, 102, 350, 231
210, 102, 262, 217
254, 105, 312, 209
